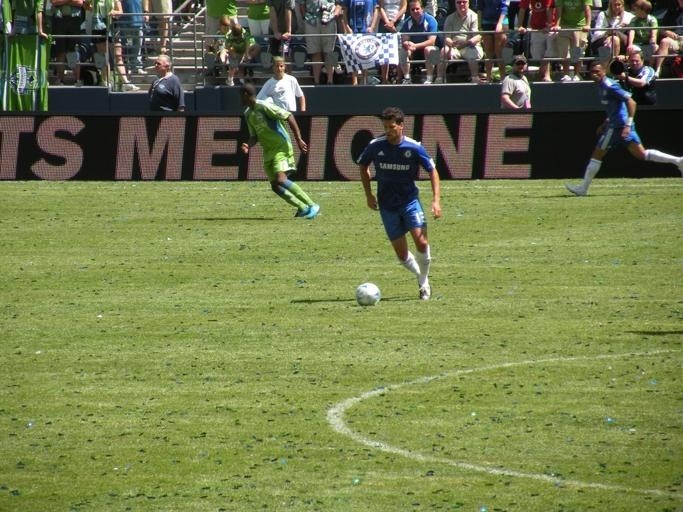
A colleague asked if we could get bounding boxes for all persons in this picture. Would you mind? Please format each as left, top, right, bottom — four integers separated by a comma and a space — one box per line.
257, 57, 306, 112
1, 0, 174, 84
237, 83, 321, 219
500, 54, 531, 110
147, 55, 186, 112
202, 0, 683, 86
357, 105, 442, 301
618, 50, 657, 105
564, 61, 683, 197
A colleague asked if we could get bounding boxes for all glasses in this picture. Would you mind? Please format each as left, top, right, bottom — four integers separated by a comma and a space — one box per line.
455, 1, 468, 4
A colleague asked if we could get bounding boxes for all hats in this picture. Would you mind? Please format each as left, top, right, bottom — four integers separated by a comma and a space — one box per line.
511, 54, 526, 65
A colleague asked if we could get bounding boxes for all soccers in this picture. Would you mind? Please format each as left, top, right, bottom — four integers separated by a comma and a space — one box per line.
356, 282, 381, 305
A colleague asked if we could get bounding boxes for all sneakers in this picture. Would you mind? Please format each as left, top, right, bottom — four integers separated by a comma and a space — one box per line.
418, 281, 430, 301
471, 75, 480, 83
560, 75, 571, 81
121, 81, 141, 92
401, 78, 412, 84
294, 206, 310, 217
572, 74, 582, 81
225, 80, 234, 87
305, 204, 319, 219
435, 77, 444, 84
424, 79, 434, 84
565, 183, 588, 197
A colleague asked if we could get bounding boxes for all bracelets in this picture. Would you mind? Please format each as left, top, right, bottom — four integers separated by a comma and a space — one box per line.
626, 116, 633, 127
